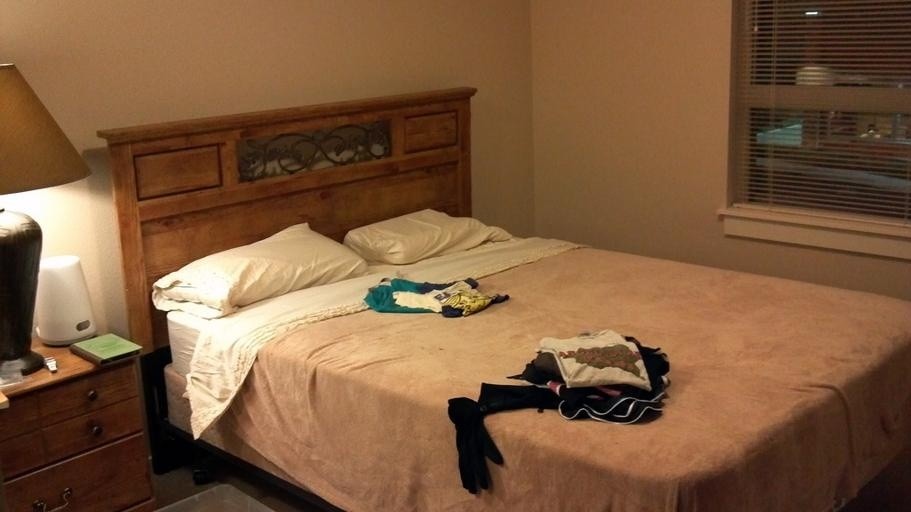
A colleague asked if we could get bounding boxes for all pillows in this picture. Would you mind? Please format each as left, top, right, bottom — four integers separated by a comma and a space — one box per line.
344, 207, 515, 265
153, 220, 372, 312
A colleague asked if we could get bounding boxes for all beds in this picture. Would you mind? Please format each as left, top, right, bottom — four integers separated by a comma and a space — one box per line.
96, 82, 911, 511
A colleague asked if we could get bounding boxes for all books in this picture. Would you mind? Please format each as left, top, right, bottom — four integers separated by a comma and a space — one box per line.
69, 332, 143, 366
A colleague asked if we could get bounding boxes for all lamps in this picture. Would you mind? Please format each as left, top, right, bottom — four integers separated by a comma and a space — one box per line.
1, 60, 92, 380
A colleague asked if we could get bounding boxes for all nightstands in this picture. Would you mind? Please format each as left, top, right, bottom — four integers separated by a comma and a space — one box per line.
0, 329, 155, 511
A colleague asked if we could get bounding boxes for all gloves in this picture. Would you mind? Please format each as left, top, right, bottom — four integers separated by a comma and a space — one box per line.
448, 397, 504, 494
